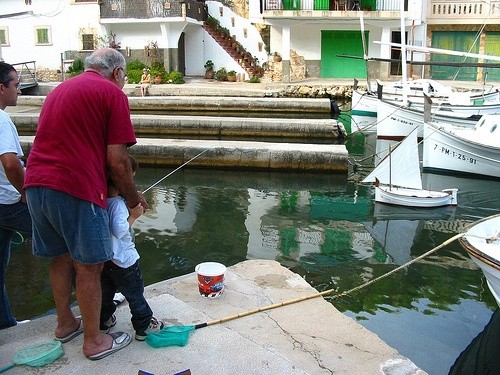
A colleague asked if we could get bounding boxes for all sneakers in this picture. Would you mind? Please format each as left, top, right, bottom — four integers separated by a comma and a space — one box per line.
100, 315, 117, 334
135, 317, 164, 341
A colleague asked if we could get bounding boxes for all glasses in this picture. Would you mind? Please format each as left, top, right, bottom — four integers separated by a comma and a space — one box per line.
8, 84, 21, 90
113, 66, 129, 81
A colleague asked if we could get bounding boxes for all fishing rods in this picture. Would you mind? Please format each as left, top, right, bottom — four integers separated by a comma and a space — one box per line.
141, 145, 213, 194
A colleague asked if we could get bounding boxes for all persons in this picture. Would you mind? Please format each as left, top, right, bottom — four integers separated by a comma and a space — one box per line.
22, 48, 137, 361
100, 151, 165, 342
139, 68, 151, 97
0, 61, 27, 329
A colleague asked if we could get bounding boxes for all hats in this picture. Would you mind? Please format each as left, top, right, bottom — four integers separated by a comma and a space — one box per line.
142, 68, 147, 72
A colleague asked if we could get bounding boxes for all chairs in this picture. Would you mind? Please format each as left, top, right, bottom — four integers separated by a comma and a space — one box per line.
351, 0, 361, 10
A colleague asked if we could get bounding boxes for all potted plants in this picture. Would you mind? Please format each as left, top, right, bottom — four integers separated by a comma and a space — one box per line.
274, 52, 282, 62
153, 63, 164, 84
204, 18, 258, 82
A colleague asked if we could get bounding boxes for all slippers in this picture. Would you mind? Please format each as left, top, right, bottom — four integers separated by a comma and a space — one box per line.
88, 332, 132, 361
54, 317, 85, 343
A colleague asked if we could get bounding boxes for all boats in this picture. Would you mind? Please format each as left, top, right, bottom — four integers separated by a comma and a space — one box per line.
349, 86, 497, 117
421, 120, 499, 182
459, 211, 500, 310
375, 97, 499, 140
375, 185, 450, 210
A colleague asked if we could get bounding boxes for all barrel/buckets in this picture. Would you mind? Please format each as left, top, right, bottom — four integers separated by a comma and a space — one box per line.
195, 262, 227, 299
